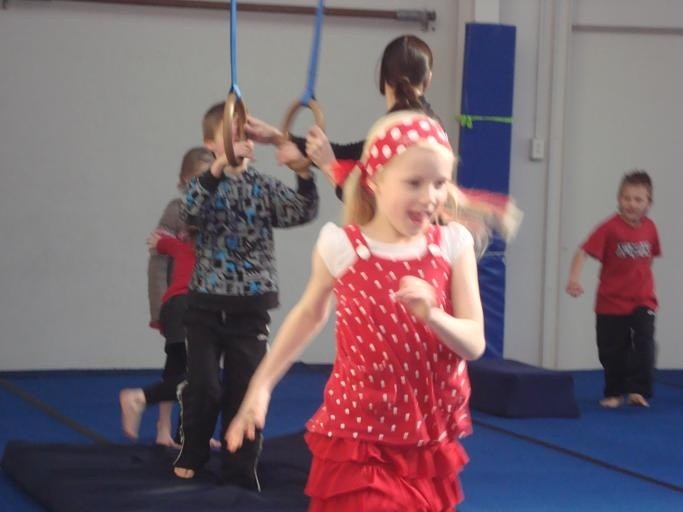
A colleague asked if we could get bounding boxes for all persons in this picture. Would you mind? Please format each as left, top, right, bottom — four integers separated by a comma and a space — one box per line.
223, 108, 487, 511
564, 169, 663, 408
146, 147, 222, 450
171, 96, 321, 489
117, 222, 195, 442
239, 32, 446, 205
